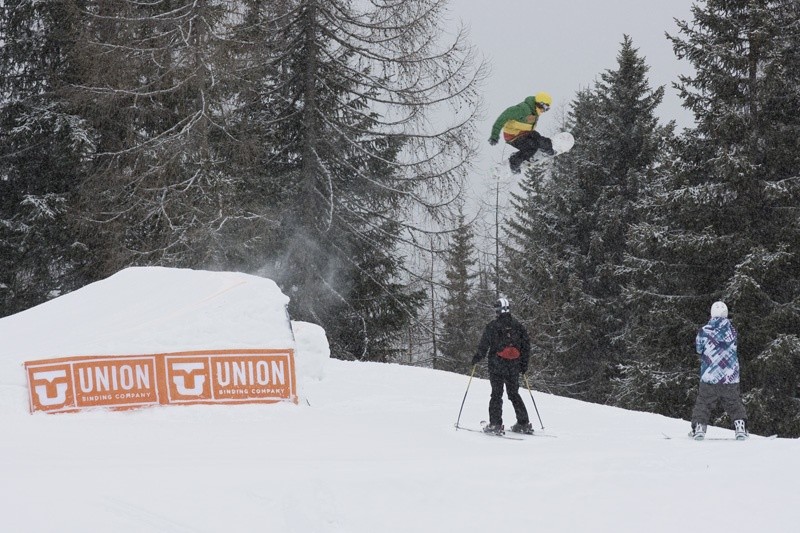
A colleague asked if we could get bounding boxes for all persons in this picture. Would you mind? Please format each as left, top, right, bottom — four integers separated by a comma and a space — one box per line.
487, 91, 557, 174
471, 298, 534, 437
687, 301, 749, 441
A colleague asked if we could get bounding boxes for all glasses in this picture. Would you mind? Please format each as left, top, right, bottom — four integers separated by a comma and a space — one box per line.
493, 304, 499, 308
535, 102, 549, 109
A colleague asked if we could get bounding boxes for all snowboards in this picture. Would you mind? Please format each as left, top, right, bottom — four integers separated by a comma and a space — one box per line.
662, 425, 780, 443
498, 131, 575, 182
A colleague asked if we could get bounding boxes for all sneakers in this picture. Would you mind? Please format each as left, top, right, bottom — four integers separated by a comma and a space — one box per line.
541, 145, 555, 156
510, 421, 534, 435
509, 155, 521, 174
482, 418, 505, 437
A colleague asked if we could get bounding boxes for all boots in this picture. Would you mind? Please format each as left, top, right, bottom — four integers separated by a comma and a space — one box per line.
733, 419, 749, 440
687, 422, 705, 439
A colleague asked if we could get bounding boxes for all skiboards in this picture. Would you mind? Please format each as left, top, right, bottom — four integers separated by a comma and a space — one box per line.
453, 419, 557, 443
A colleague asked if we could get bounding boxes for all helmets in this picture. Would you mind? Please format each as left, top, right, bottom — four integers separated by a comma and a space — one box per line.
710, 301, 728, 318
493, 298, 510, 314
535, 91, 553, 106
